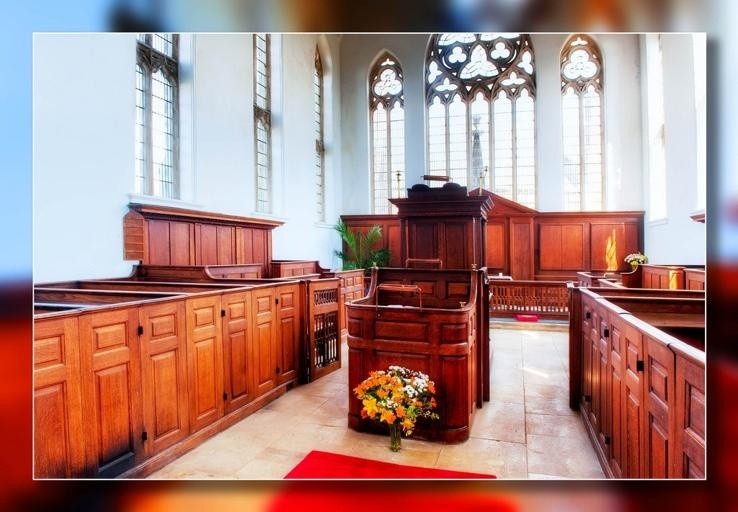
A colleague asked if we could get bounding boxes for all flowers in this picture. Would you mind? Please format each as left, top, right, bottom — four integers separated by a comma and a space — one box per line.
352, 365, 439, 438
623, 251, 647, 264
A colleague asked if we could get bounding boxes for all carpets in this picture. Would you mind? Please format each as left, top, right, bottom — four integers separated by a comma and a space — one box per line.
283, 450, 498, 480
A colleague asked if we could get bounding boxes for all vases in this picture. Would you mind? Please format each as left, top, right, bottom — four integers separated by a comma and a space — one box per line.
389, 424, 403, 451
630, 261, 638, 272
